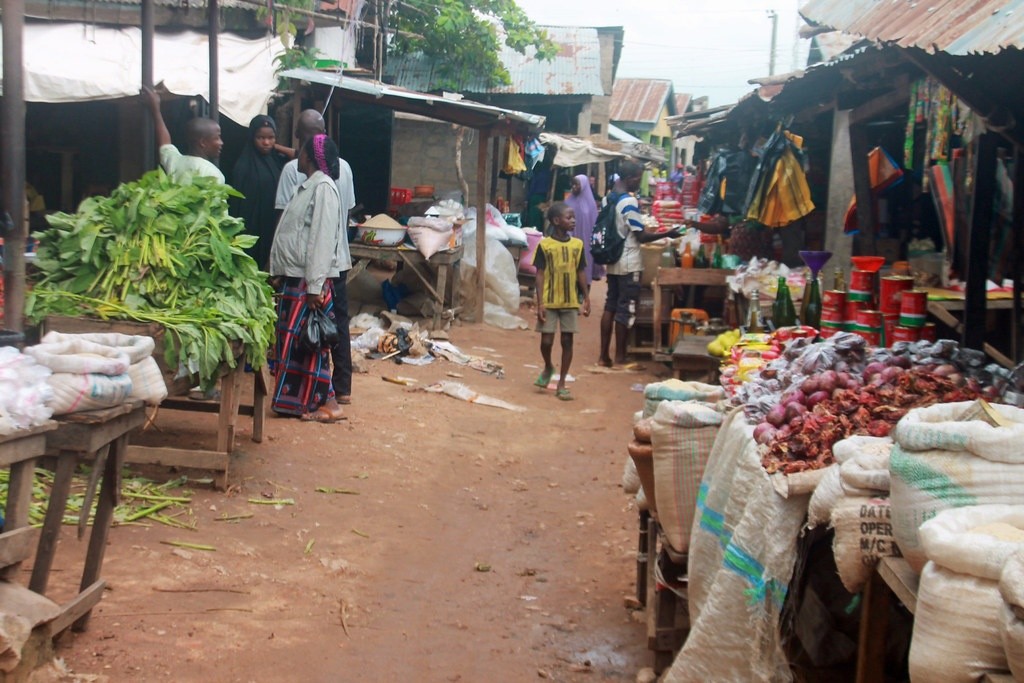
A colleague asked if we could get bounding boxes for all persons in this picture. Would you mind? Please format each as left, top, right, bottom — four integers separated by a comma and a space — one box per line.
230, 115, 300, 375
532, 202, 591, 401
270, 134, 348, 421
684, 212, 728, 233
531, 170, 619, 302
640, 164, 695, 197
597, 160, 684, 369
139, 87, 225, 400
273, 110, 355, 405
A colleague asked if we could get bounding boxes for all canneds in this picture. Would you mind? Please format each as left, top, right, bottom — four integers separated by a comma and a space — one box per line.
820, 269, 936, 347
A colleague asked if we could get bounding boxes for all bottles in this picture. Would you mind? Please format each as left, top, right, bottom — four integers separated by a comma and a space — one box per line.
681, 242, 693, 268
799, 279, 812, 325
661, 239, 673, 268
804, 279, 822, 331
746, 289, 763, 333
772, 277, 796, 329
834, 271, 845, 290
693, 244, 707, 269
711, 245, 723, 268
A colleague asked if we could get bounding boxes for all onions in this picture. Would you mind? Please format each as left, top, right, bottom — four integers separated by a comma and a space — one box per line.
753, 355, 998, 445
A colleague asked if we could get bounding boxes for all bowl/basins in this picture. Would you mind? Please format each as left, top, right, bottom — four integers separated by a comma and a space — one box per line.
850, 255, 886, 273
356, 226, 408, 246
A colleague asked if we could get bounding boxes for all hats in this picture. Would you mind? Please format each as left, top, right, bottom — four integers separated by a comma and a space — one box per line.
312, 134, 340, 181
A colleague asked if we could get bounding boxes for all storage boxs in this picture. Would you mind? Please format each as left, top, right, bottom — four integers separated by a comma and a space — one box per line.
391, 186, 412, 210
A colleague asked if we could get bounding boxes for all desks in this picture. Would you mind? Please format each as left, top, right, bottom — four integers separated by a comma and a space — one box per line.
348, 244, 463, 335
916, 289, 1016, 371
38, 309, 268, 489
855, 556, 1017, 683
673, 334, 718, 384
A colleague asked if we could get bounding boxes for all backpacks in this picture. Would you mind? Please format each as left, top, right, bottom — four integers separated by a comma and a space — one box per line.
589, 192, 630, 264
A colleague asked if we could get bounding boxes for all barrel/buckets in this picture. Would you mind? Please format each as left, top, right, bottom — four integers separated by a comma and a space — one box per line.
818, 270, 935, 347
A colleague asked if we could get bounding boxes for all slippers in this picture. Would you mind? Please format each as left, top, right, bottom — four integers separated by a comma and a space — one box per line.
534, 367, 555, 389
595, 360, 614, 368
335, 395, 351, 404
301, 406, 347, 423
557, 389, 573, 400
612, 362, 646, 371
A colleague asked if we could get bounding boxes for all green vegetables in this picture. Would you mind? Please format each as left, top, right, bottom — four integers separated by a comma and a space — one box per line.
18, 164, 278, 397
